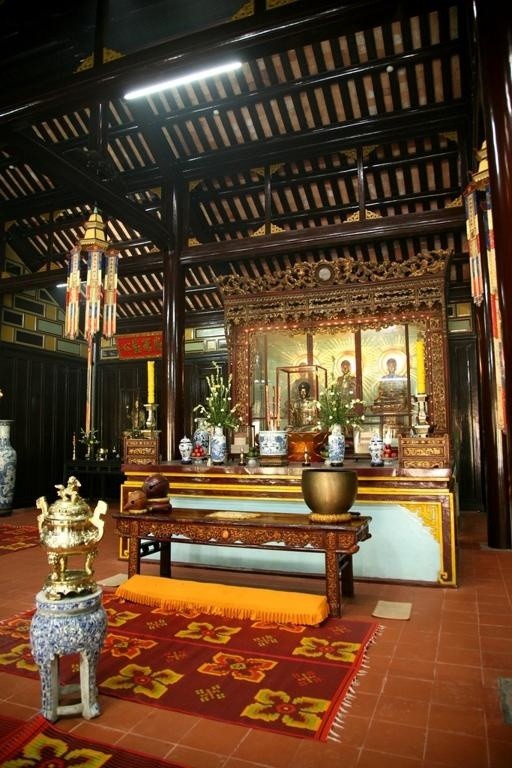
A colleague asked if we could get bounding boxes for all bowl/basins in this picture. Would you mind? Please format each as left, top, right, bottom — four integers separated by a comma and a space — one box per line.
258, 429, 288, 457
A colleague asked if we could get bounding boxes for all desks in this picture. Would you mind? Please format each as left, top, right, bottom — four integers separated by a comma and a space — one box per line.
113, 508, 372, 618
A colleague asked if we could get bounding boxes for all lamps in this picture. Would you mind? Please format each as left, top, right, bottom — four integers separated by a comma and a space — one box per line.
65, 207, 118, 459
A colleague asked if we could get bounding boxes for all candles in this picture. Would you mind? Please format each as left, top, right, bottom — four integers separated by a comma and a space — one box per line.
415, 341, 426, 394
147, 360, 155, 404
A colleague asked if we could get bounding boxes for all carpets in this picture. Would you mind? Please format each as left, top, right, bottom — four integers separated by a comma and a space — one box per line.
0, 586, 385, 743
0, 715, 190, 768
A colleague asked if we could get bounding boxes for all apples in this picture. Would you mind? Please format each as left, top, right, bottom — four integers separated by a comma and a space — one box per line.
383, 444, 396, 458
192, 445, 205, 457
247, 447, 258, 457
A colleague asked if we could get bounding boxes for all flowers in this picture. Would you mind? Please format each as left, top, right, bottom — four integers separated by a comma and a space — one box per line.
194, 361, 244, 432
304, 379, 366, 431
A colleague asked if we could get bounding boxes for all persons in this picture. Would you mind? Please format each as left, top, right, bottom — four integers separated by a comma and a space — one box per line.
284, 363, 307, 406
380, 358, 406, 410
337, 360, 354, 410
289, 382, 323, 428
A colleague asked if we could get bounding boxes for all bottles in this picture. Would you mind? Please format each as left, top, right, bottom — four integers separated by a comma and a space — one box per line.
179, 435, 192, 462
192, 418, 210, 456
369, 432, 384, 465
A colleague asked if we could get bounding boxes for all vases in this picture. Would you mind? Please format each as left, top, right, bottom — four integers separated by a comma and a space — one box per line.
210, 426, 227, 463
328, 425, 346, 463
